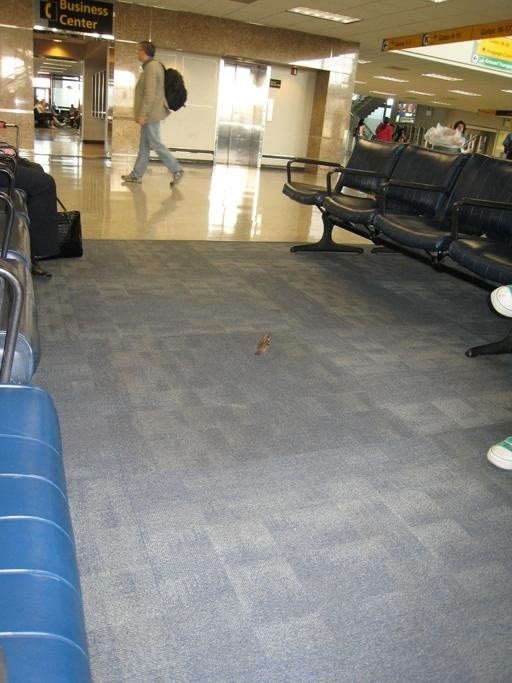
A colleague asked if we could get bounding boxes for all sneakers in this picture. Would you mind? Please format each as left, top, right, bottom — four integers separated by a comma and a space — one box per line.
30, 263, 53, 279
488, 282, 512, 319
486, 433, 512, 472
169, 168, 185, 186
120, 171, 143, 182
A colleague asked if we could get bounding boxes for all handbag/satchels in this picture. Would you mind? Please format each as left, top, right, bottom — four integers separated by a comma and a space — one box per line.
31, 197, 84, 261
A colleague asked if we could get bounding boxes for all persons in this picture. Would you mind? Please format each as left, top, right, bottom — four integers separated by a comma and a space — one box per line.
485, 281, 512, 479
35, 100, 66, 127
377, 115, 395, 141
357, 117, 364, 135
122, 39, 188, 187
451, 119, 467, 142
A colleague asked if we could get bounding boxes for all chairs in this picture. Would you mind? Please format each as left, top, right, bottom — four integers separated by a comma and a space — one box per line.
281, 137, 511, 358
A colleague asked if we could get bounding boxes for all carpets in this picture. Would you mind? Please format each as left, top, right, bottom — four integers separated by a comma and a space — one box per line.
28, 239, 512, 681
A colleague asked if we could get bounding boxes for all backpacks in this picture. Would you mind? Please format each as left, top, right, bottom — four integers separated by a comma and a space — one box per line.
159, 59, 188, 112
